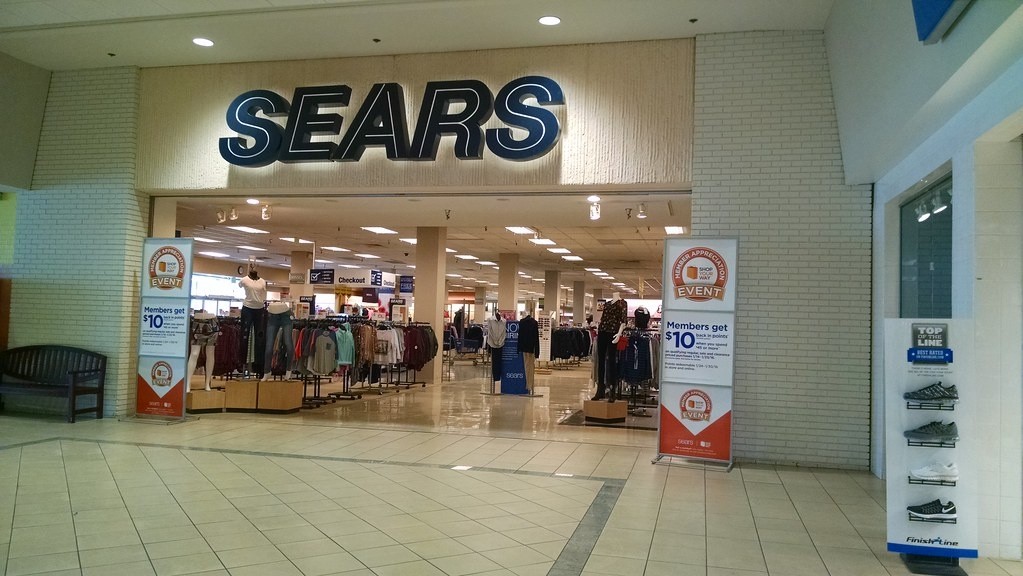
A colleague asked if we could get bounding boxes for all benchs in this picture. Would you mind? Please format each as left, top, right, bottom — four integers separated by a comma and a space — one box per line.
0, 345, 108, 423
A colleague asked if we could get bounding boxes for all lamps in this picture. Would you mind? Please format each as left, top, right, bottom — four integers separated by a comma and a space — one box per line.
636, 204, 647, 218
217, 210, 226, 223
229, 209, 238, 220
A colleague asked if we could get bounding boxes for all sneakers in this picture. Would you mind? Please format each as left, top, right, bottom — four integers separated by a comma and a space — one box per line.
910, 462, 959, 482
903, 421, 959, 442
902, 380, 960, 404
907, 499, 958, 519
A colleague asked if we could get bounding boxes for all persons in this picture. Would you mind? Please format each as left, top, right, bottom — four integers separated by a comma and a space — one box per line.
186, 312, 219, 392
517, 314, 539, 389
591, 291, 628, 403
262, 302, 295, 383
486, 311, 506, 381
237, 271, 267, 366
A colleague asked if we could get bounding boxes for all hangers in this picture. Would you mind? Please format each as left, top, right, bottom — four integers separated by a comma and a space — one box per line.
559, 325, 659, 339
292, 303, 432, 331
217, 317, 241, 325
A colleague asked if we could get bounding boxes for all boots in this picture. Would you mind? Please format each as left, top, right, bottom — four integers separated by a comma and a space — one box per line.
591, 382, 617, 403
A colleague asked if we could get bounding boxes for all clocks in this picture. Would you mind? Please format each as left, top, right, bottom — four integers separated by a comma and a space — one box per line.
238, 266, 244, 274
